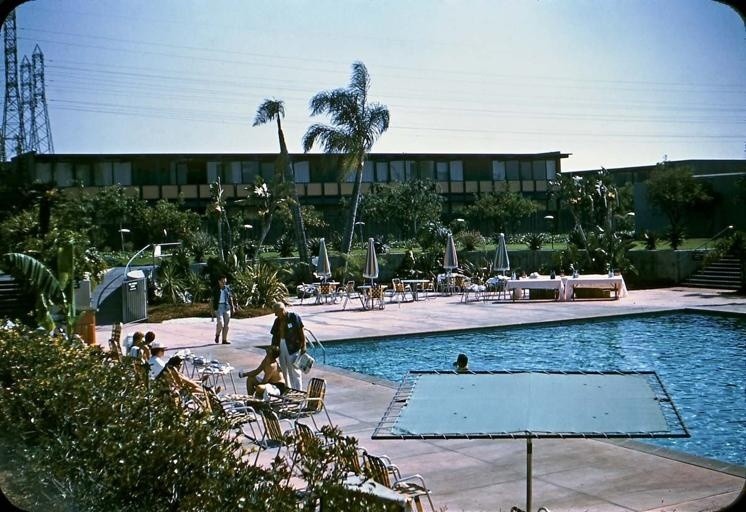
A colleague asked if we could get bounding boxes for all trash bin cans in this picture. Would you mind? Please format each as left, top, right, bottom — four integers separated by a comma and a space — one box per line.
77, 310, 96, 344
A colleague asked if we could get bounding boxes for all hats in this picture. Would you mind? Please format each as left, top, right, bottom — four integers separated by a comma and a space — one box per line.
151, 342, 167, 349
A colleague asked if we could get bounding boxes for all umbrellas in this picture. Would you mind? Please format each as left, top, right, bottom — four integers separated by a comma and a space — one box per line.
317, 237, 332, 303
362, 237, 379, 309
493, 233, 511, 298
443, 233, 458, 294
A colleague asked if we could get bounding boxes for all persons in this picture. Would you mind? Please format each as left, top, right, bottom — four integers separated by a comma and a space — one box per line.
271, 301, 307, 394
137, 331, 156, 365
156, 356, 220, 396
239, 344, 281, 399
454, 353, 470, 372
149, 339, 167, 380
210, 274, 235, 344
128, 332, 144, 356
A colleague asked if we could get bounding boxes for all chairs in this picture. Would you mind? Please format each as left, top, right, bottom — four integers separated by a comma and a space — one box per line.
300, 271, 527, 311
104, 319, 436, 512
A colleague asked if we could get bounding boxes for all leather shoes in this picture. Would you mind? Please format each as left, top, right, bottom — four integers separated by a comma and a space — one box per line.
215, 335, 219, 343
222, 342, 230, 344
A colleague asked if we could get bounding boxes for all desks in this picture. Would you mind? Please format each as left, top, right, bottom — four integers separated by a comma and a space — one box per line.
507, 274, 623, 303
